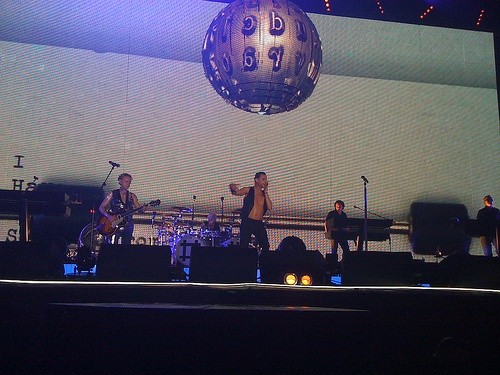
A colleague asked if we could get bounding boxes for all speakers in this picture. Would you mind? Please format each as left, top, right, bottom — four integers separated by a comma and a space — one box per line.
188, 247, 258, 283
0, 240, 67, 280
436, 255, 500, 289
344, 250, 412, 287
258, 251, 326, 285
99, 244, 170, 282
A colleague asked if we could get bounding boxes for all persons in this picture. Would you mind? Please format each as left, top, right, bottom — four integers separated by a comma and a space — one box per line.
324, 200, 350, 255
230, 172, 273, 252
100, 174, 150, 245
201, 212, 220, 244
0, 271, 500, 375
477, 195, 500, 257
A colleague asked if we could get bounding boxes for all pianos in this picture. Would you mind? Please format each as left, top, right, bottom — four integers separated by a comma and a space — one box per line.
324, 217, 393, 252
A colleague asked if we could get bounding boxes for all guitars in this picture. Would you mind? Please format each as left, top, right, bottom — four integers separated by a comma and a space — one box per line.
97, 199, 161, 237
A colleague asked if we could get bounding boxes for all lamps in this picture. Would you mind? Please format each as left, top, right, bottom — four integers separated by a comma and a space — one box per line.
283, 272, 298, 285
299, 272, 313, 286
202, 0, 323, 116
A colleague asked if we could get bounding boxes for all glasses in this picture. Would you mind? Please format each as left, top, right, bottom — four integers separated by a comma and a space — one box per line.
484, 199, 493, 201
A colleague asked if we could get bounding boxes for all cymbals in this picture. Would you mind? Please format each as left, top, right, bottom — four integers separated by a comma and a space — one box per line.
171, 206, 193, 212
231, 209, 241, 213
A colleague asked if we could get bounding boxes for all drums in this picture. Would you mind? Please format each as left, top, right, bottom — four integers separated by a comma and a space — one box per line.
81, 223, 105, 253
174, 225, 255, 266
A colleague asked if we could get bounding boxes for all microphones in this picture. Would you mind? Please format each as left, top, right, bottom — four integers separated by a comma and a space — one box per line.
360, 176, 369, 184
109, 161, 120, 167
